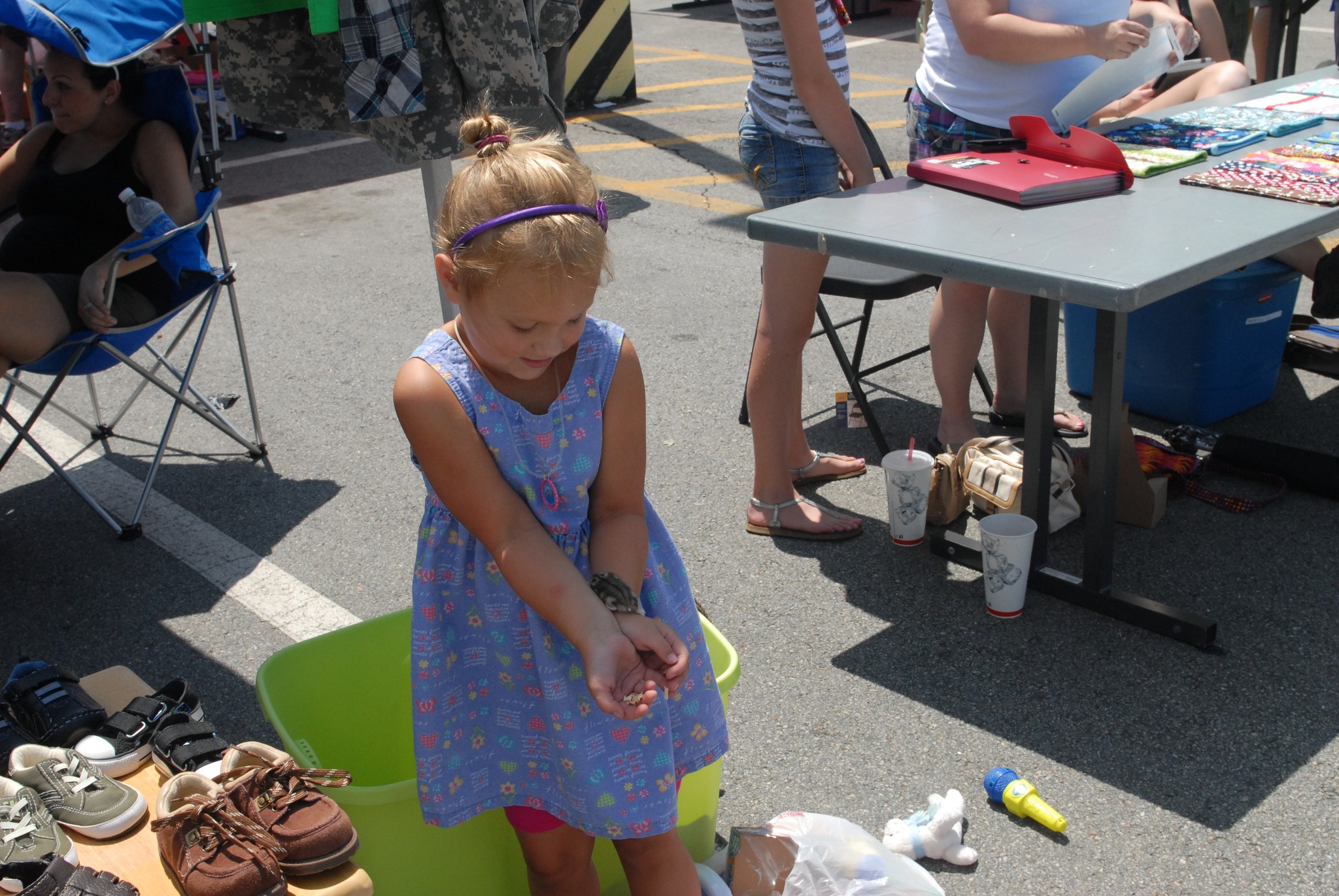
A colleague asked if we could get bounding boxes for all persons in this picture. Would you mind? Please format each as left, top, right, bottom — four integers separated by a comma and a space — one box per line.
0, 45, 197, 379
393, 116, 728, 896
1088, 0, 1339, 319
906, 0, 1193, 457
732, 0, 876, 539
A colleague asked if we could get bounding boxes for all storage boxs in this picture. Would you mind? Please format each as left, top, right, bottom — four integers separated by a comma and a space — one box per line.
1064, 257, 1302, 427
255, 601, 742, 896
835, 392, 868, 428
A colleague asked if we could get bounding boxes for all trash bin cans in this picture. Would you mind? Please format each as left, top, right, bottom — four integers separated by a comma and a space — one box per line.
256, 607, 741, 896
1064, 258, 1302, 428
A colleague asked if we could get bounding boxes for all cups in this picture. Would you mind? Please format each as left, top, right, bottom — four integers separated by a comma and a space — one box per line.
881, 449, 936, 548
979, 514, 1036, 619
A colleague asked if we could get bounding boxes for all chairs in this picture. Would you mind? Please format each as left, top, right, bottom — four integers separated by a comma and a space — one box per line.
0, 0, 268, 568
736, 106, 994, 427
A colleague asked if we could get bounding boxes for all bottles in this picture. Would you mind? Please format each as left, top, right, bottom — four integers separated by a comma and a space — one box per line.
118, 188, 181, 240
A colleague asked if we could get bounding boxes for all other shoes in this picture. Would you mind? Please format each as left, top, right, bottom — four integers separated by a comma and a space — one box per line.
1311, 244, 1339, 319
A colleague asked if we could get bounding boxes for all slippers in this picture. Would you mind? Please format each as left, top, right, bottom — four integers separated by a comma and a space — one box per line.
988, 402, 1088, 438
928, 432, 947, 454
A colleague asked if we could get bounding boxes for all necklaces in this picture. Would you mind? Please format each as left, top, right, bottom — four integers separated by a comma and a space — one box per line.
454, 313, 565, 510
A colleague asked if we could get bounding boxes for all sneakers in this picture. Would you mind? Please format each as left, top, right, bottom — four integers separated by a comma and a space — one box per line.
0, 776, 79, 867
212, 742, 360, 876
0, 655, 108, 749
149, 711, 229, 787
9, 744, 148, 839
0, 698, 39, 776
151, 772, 288, 896
74, 677, 204, 778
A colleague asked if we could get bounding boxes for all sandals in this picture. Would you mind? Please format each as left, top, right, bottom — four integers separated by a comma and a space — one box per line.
787, 450, 866, 486
0, 852, 140, 896
745, 496, 863, 540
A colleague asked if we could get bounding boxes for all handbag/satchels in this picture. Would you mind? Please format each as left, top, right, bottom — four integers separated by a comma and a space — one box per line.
761, 811, 945, 896
958, 435, 1081, 534
926, 453, 970, 524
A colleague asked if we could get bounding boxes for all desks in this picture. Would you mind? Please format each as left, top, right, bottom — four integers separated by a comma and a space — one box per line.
746, 65, 1339, 658
